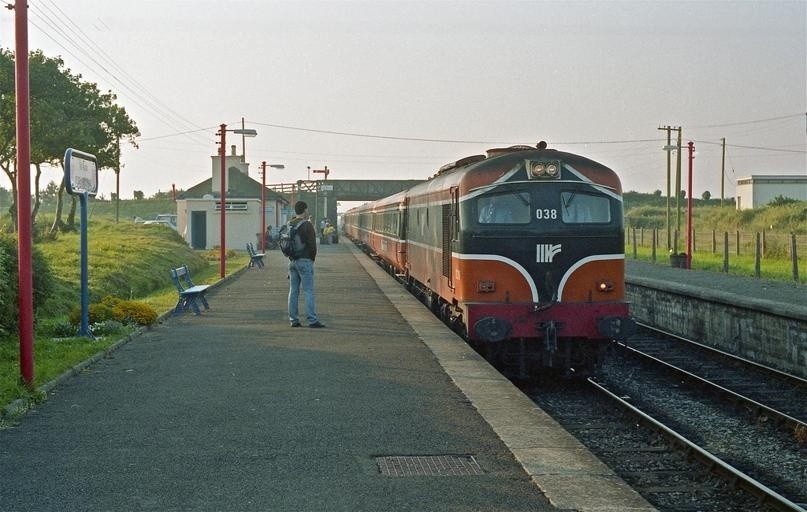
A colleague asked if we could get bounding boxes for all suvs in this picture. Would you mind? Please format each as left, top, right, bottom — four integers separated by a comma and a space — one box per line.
151, 215, 177, 226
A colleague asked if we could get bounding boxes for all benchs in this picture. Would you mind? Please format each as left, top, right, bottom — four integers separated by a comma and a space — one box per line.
246, 243, 265, 268
170, 264, 211, 314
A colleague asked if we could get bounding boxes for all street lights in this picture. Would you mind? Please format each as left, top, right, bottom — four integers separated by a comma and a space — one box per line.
662, 140, 696, 272
258, 160, 285, 253
216, 123, 257, 280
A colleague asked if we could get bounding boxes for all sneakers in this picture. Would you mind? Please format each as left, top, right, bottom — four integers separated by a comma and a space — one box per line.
309, 321, 325, 327
291, 322, 301, 326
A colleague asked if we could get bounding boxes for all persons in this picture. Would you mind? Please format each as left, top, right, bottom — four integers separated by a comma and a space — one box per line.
286, 201, 325, 328
320, 217, 336, 244
478, 196, 512, 224
561, 193, 592, 223
266, 224, 280, 250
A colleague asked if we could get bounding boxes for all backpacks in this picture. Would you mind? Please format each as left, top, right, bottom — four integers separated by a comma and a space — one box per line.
279, 220, 308, 256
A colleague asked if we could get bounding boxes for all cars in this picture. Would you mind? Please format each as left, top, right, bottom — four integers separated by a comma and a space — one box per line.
143, 220, 176, 231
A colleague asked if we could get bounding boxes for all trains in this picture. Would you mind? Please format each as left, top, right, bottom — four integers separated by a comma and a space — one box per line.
339, 139, 633, 392
